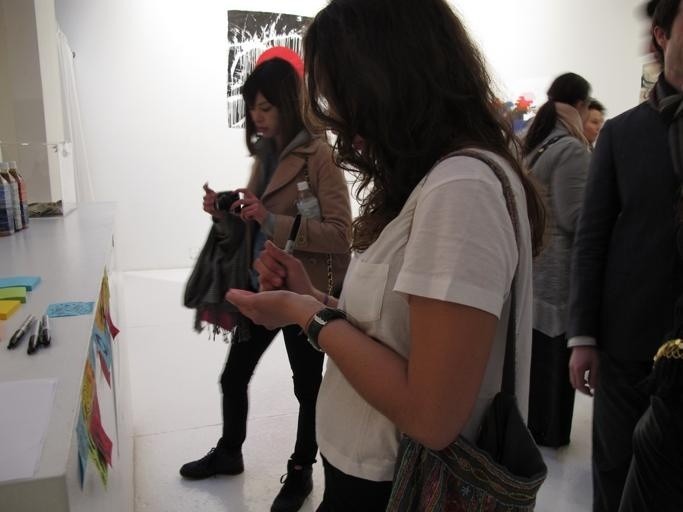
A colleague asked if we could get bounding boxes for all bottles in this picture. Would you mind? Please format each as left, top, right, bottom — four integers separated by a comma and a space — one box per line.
0, 162, 27, 236
294, 181, 322, 218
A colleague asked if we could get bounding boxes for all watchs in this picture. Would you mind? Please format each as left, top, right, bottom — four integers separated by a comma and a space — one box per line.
306, 307, 355, 354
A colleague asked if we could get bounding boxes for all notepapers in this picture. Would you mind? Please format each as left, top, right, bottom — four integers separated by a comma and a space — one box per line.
0, 276, 40, 320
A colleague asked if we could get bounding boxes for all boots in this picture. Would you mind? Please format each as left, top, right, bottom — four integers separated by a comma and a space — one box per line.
180, 439, 244, 479
271, 460, 314, 511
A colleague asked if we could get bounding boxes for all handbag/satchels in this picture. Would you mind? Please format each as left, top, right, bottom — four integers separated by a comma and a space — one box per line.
384, 393, 548, 510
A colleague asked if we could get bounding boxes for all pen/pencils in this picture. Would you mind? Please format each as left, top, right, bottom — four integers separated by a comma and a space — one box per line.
285, 215, 301, 255
7, 314, 51, 355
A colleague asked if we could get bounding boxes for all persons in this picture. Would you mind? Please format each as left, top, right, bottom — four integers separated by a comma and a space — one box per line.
222, 2, 546, 511
180, 58, 353, 512
519, 0, 681, 512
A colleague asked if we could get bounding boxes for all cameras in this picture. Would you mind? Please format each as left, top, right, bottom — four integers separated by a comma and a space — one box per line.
214, 191, 242, 214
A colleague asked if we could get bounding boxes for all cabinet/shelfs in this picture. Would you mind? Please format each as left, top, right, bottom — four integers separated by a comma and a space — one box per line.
2, 199, 137, 512
